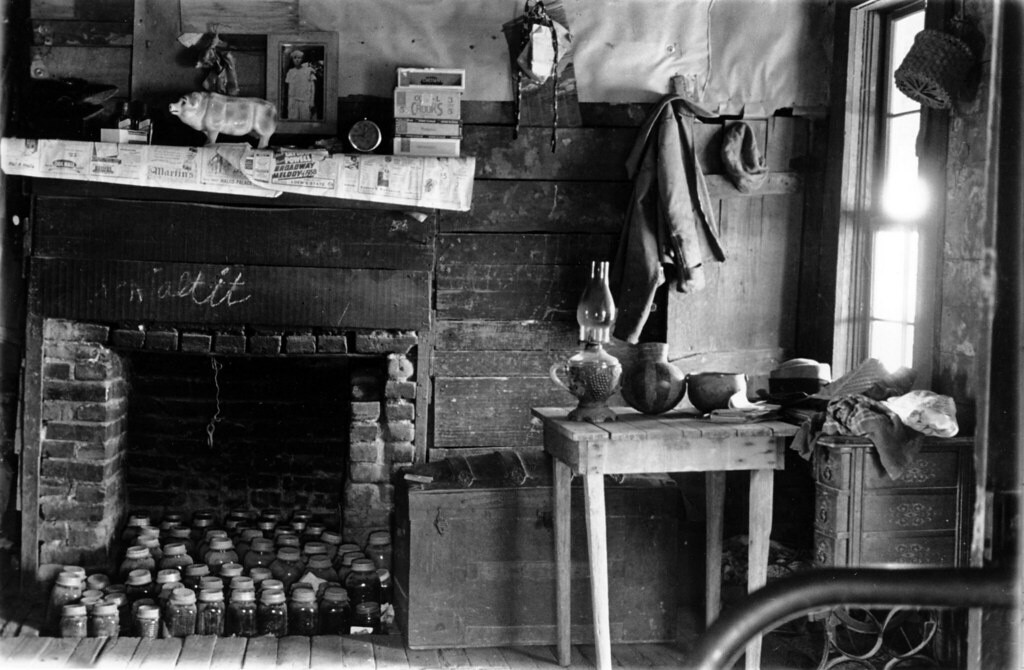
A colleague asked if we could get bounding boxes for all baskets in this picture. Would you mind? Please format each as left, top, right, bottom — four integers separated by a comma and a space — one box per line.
895, 29, 976, 110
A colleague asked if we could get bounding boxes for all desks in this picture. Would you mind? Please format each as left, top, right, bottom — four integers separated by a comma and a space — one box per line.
530, 406, 801, 670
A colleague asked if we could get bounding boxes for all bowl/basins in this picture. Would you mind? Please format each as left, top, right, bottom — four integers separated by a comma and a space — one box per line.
688, 372, 745, 413
768, 378, 829, 395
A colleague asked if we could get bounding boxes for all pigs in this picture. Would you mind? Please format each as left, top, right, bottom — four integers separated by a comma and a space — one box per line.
168, 91, 279, 149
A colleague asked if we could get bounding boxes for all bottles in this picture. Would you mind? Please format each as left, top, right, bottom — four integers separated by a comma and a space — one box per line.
44, 509, 393, 638
622, 343, 687, 417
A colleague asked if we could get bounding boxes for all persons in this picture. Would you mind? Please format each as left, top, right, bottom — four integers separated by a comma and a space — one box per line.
285, 51, 316, 120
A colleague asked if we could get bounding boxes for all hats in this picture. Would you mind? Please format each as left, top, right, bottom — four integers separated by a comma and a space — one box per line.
291, 50, 304, 58
720, 120, 769, 194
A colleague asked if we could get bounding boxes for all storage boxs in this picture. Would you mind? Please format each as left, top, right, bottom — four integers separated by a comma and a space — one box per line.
393, 84, 464, 120
398, 68, 468, 90
395, 119, 464, 138
100, 127, 148, 144
394, 138, 460, 158
394, 486, 681, 651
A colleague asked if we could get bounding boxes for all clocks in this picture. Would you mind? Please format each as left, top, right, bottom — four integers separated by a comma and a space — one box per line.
348, 115, 383, 152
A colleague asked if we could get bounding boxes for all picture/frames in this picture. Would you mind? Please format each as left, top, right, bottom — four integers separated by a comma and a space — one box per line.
264, 32, 341, 135
